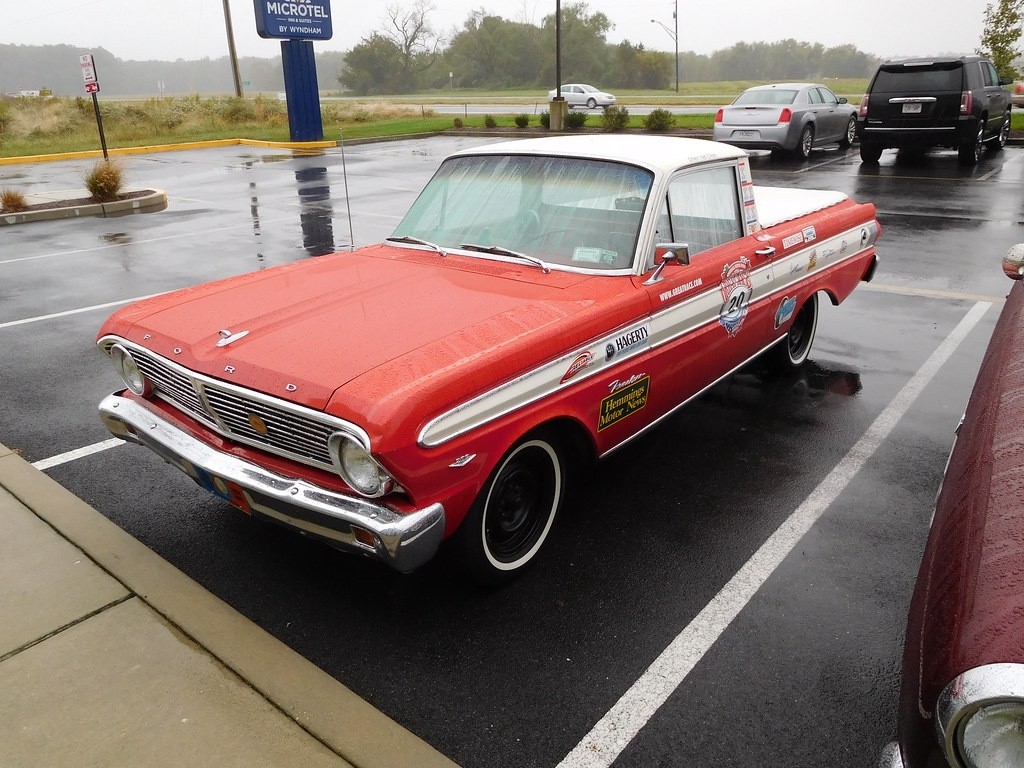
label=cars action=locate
[712,82,859,162]
[94,132,882,587]
[876,239,1023,768]
[548,84,616,110]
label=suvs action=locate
[855,54,1012,171]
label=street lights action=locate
[651,19,680,93]
